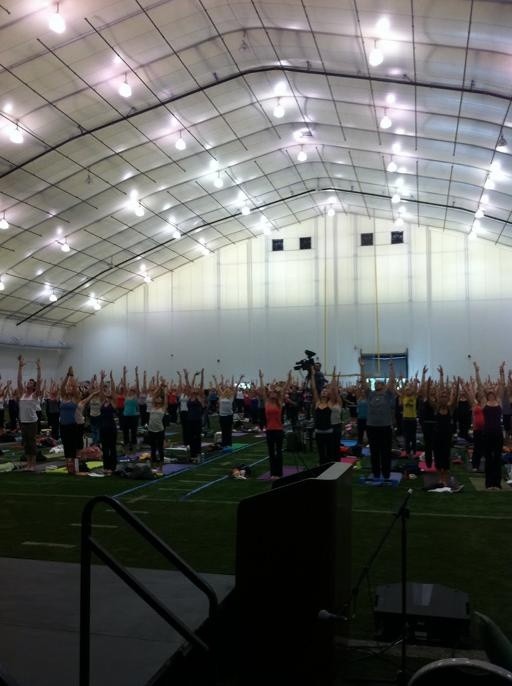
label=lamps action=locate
[213,170,272,237]
[380,108,391,128]
[49,2,67,34]
[173,225,180,239]
[10,122,24,143]
[202,243,210,257]
[85,170,93,185]
[387,141,412,228]
[60,237,71,254]
[239,29,251,54]
[367,37,383,67]
[144,272,152,284]
[297,145,308,163]
[93,299,101,310]
[119,72,132,98]
[1,279,5,291]
[315,179,337,216]
[467,162,500,238]
[106,256,115,269]
[49,289,57,302]
[2,210,9,230]
[135,199,144,217]
[272,98,285,119]
[496,132,508,151]
[175,130,186,150]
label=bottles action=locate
[74,458,80,473]
[439,467,445,481]
[200,452,206,464]
[464,452,467,464]
[262,425,268,441]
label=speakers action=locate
[236,461,353,629]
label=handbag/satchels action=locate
[132,465,153,480]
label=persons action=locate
[0,348,511,493]
